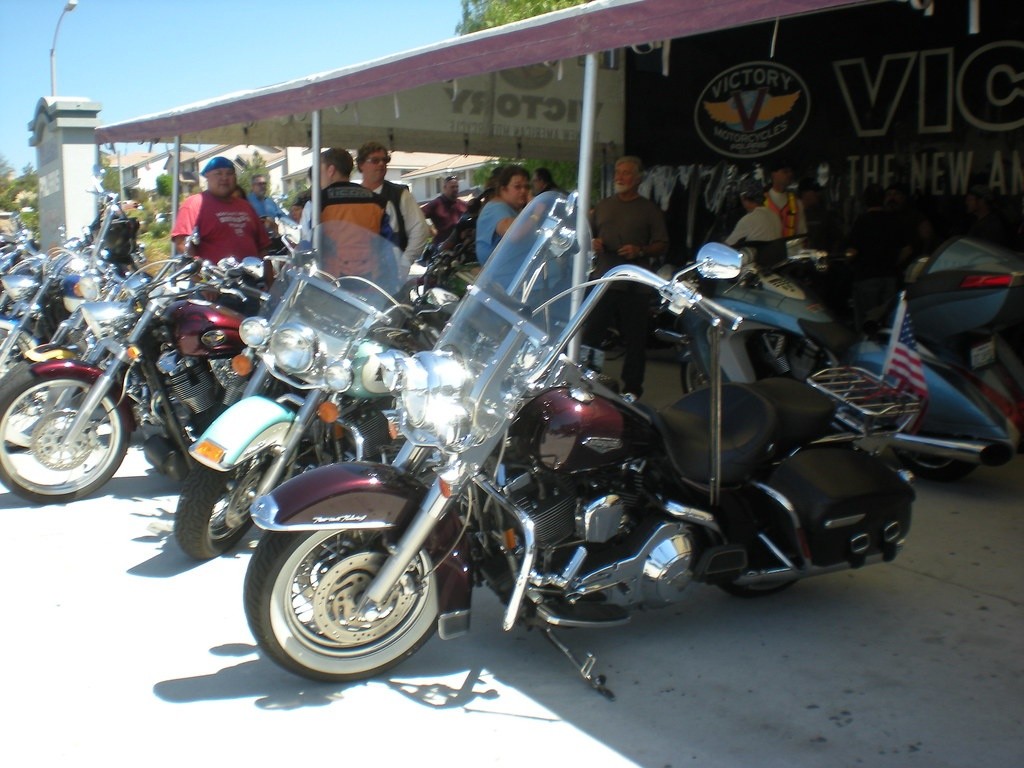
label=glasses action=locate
[255,182,266,185]
[367,156,390,164]
[446,176,457,181]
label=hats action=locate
[200,157,233,176]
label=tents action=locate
[96,1,1024,368]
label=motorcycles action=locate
[0,191,271,504]
[652,236,1024,483]
[173,221,460,562]
[243,189,927,704]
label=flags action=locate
[881,297,931,437]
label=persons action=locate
[356,141,428,310]
[289,166,313,224]
[248,173,285,225]
[290,203,304,223]
[297,146,402,297]
[726,164,950,292]
[420,164,596,283]
[584,153,668,400]
[169,157,266,317]
[231,185,248,200]
[476,164,562,335]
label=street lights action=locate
[50,0,78,97]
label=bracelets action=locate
[591,238,595,242]
[530,215,539,221]
